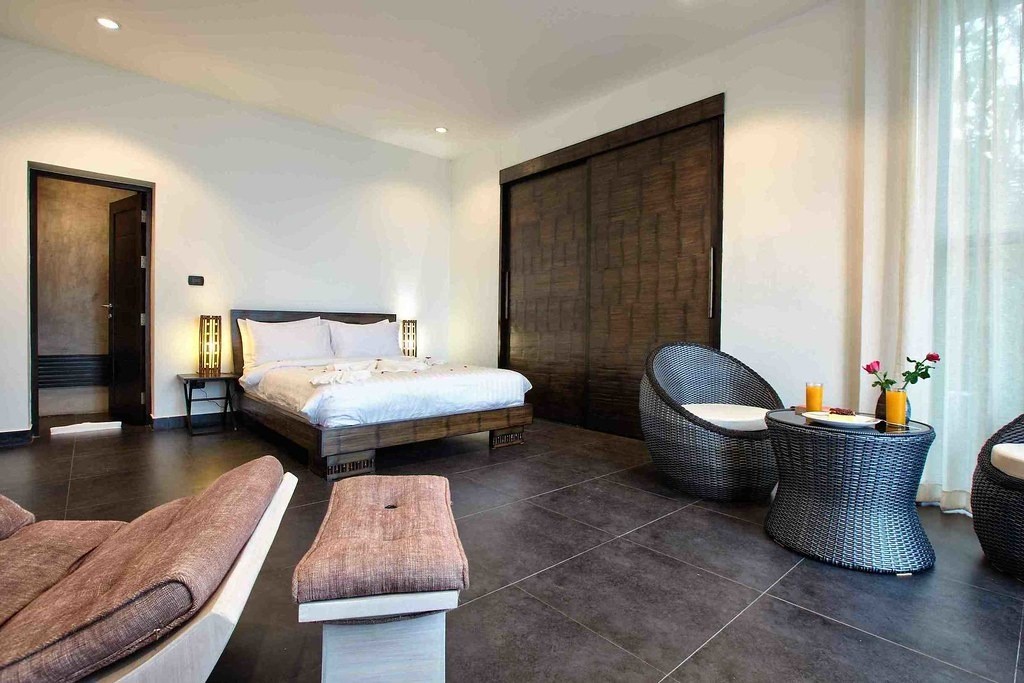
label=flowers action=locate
[861,352,940,390]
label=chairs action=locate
[0,456,297,683]
[970,414,1024,574]
[639,341,784,499]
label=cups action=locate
[886,388,906,427]
[806,382,823,412]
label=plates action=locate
[802,412,880,428]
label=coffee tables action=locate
[764,406,937,576]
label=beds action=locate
[229,309,535,482]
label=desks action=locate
[177,374,241,437]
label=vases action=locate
[875,390,910,431]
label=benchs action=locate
[291,474,469,683]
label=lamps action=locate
[199,314,222,379]
[402,319,417,357]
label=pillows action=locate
[235,316,321,369]
[323,318,390,360]
[329,321,402,360]
[245,316,332,367]
